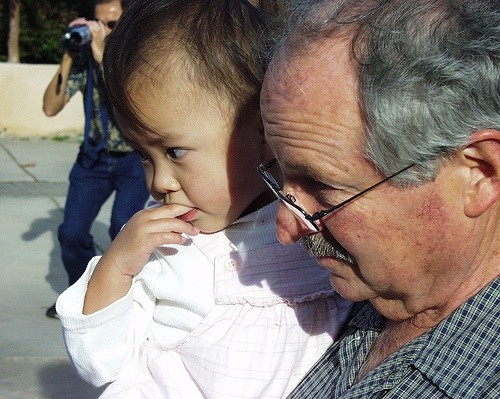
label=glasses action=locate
[255,160,416,233]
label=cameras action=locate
[65,24,92,48]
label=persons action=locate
[56,1,352,399]
[42,1,150,317]
[258,0,499,399]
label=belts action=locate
[101,149,130,157]
